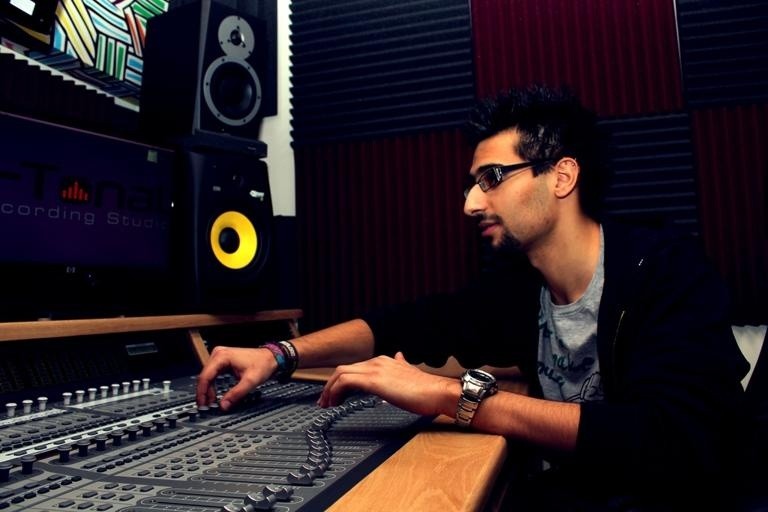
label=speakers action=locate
[139,0,268,159]
[186,151,274,315]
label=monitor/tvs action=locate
[0,108,180,269]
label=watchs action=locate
[453,368,499,429]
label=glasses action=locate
[463,157,557,199]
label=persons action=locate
[195,79,751,512]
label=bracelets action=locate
[257,340,298,385]
